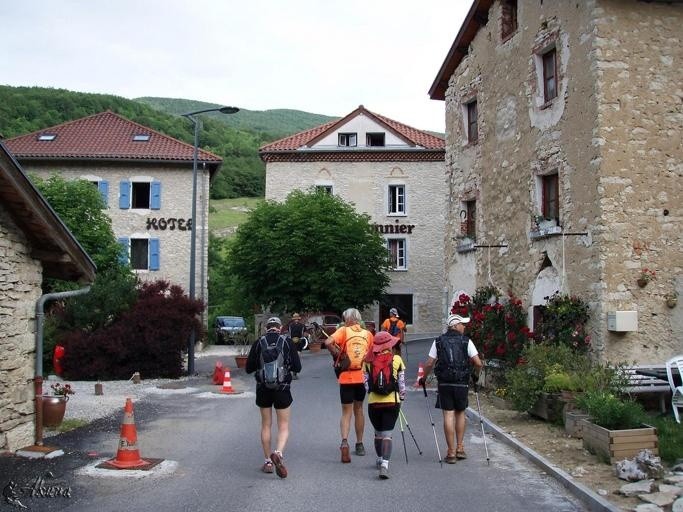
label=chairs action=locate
[665,354,683,426]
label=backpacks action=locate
[258,334,289,392]
[440,331,474,387]
[363,352,398,395]
[339,327,368,372]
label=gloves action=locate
[419,377,425,385]
[471,373,479,383]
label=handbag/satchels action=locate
[333,326,351,378]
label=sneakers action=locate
[264,462,273,473]
[456,448,468,460]
[340,443,352,463]
[269,453,288,478]
[444,455,456,464]
[377,458,382,469]
[292,375,298,380]
[379,466,390,479]
[355,446,366,456]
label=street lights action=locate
[180,105,241,377]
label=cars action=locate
[281,311,376,345]
[212,315,249,344]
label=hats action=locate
[446,315,471,326]
[291,313,302,321]
[372,332,400,353]
[267,317,282,326]
[390,308,401,318]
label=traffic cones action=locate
[100,396,151,470]
[219,367,234,392]
[413,363,428,386]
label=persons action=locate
[381,308,407,358]
[289,313,307,380]
[417,314,482,463]
[243,316,303,477]
[322,308,372,463]
[361,331,407,478]
